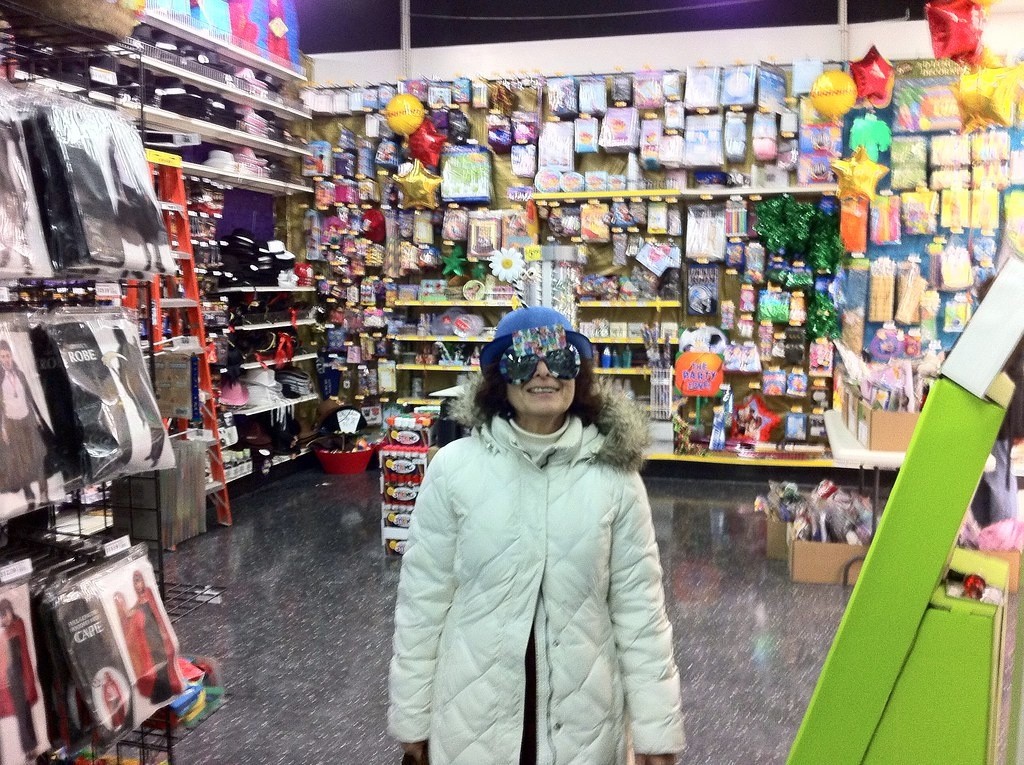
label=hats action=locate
[201,144,273,179]
[257,71,279,92]
[193,49,227,71]
[311,399,341,429]
[240,411,319,455]
[131,24,157,46]
[221,232,297,288]
[154,76,280,141]
[179,44,197,62]
[247,364,311,396]
[234,67,256,84]
[155,33,186,56]
[480,306,594,386]
[218,379,249,406]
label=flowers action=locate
[488,248,525,282]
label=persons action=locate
[388,306,686,765]
[0,340,60,511]
[0,599,38,761]
[114,571,181,705]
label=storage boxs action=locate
[842,391,921,450]
[789,539,871,586]
[767,520,792,560]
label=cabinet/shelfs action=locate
[116,5,322,494]
[394,182,834,467]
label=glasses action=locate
[500,343,582,385]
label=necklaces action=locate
[7,375,18,398]
